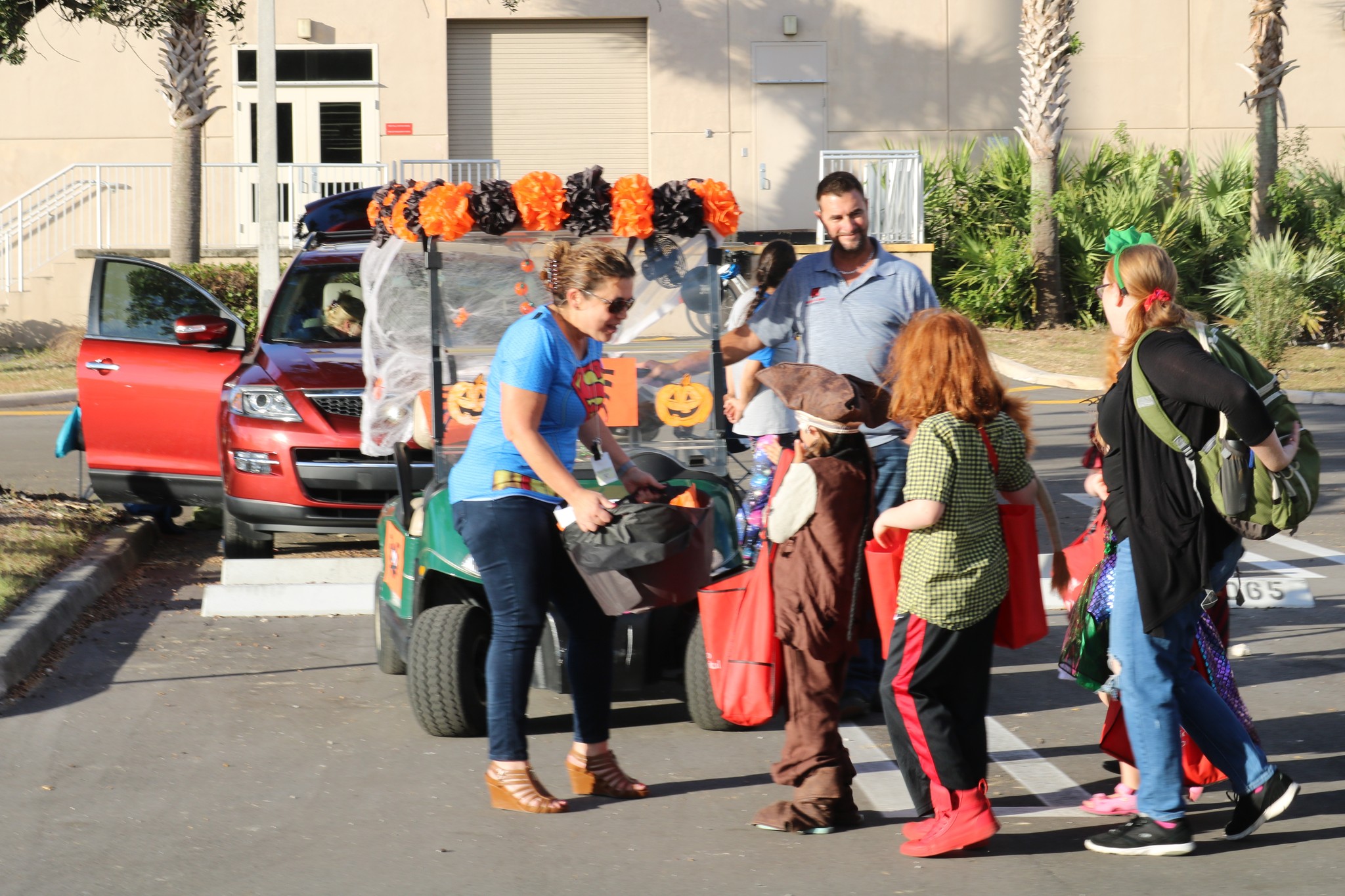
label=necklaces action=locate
[557,307,603,461]
[837,247,876,275]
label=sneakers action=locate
[899,779,999,855]
[1085,815,1195,857]
[900,815,936,841]
[1225,767,1301,840]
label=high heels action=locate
[567,747,648,799]
[485,761,568,813]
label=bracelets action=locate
[615,460,638,480]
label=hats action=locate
[757,363,869,434]
[338,294,365,320]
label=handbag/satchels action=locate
[697,448,794,725]
[863,424,1050,659]
[1053,501,1108,611]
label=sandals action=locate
[1080,784,1141,817]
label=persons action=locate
[448,241,664,814]
[286,293,366,343]
[636,172,940,724]
[1058,226,1300,857]
[871,311,1041,857]
[751,362,892,834]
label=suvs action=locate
[77,227,434,561]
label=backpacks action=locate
[1128,324,1319,540]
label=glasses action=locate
[1096,283,1124,299]
[569,284,636,312]
